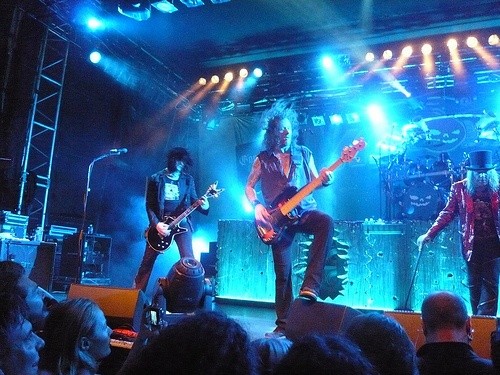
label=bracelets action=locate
[250,199,260,209]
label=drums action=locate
[429,159,452,186]
[401,182,448,223]
[403,161,429,186]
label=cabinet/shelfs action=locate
[59,234,113,279]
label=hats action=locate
[464,151,497,170]
[169,147,192,164]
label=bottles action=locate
[88,224,94,235]
[34,227,42,242]
[363,217,385,225]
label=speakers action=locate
[287,299,364,343]
[67,283,149,332]
[0,238,57,293]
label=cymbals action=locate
[377,153,403,164]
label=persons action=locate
[244,104,336,338]
[415,150,500,320]
[0,260,497,375]
[131,147,210,294]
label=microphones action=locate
[109,148,128,154]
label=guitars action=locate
[145,177,226,254]
[254,138,366,244]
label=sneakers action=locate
[265,326,286,339]
[299,286,318,301]
